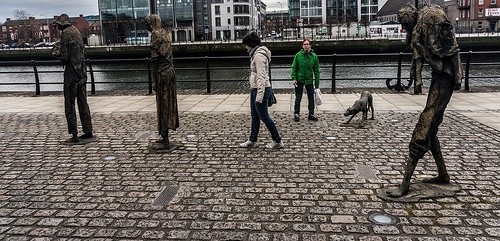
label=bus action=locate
[271,31,275,35]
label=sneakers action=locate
[240,140,258,147]
[265,140,284,149]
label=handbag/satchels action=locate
[314,89,322,104]
[268,92,276,107]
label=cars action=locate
[266,33,271,37]
[0,42,56,48]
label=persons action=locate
[240,34,284,148]
[386,4,455,197]
[53,14,93,142]
[144,14,179,149]
[291,39,320,121]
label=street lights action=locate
[40,14,50,42]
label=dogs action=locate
[343,92,375,126]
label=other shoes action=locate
[308,114,318,121]
[294,114,300,120]
